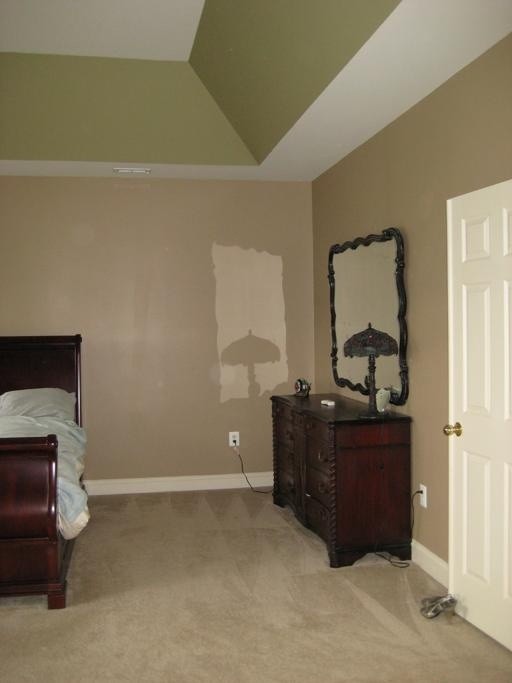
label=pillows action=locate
[0,388,77,419]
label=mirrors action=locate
[328,227,408,406]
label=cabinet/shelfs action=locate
[269,393,414,568]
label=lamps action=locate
[344,322,399,420]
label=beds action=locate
[0,335,82,609]
